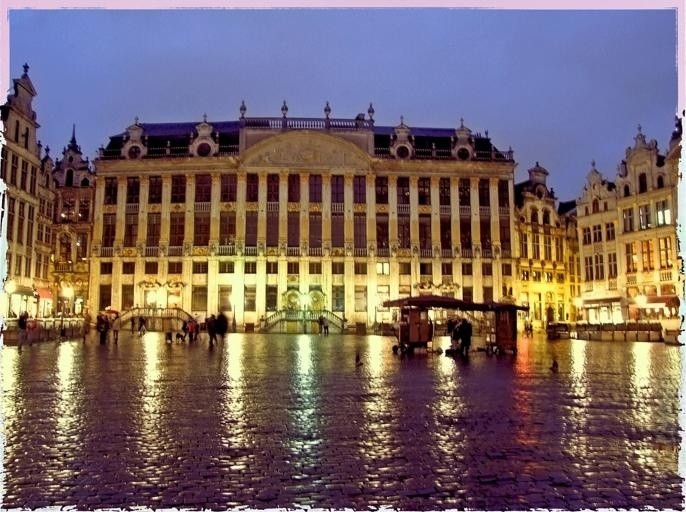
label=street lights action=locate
[298,293,309,334]
[371,292,381,322]
[227,292,243,333]
[59,284,74,343]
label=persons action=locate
[97,312,227,344]
[319,314,323,333]
[323,317,329,334]
[18,312,28,350]
[458,318,473,364]
